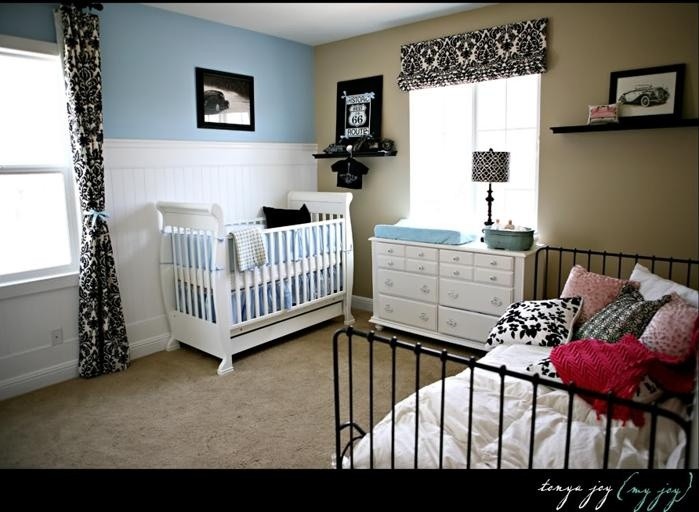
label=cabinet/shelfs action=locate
[367,235,548,352]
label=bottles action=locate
[504,220,516,230]
[491,218,503,229]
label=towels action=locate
[230,227,272,273]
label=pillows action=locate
[559,264,640,320]
[261,204,312,229]
[638,290,699,365]
[572,282,674,345]
[484,296,584,349]
[625,262,699,308]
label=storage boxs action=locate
[480,222,536,253]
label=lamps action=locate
[472,147,511,245]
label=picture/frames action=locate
[194,66,256,132]
[609,62,687,118]
[337,75,383,145]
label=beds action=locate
[152,190,355,375]
[331,245,698,470]
